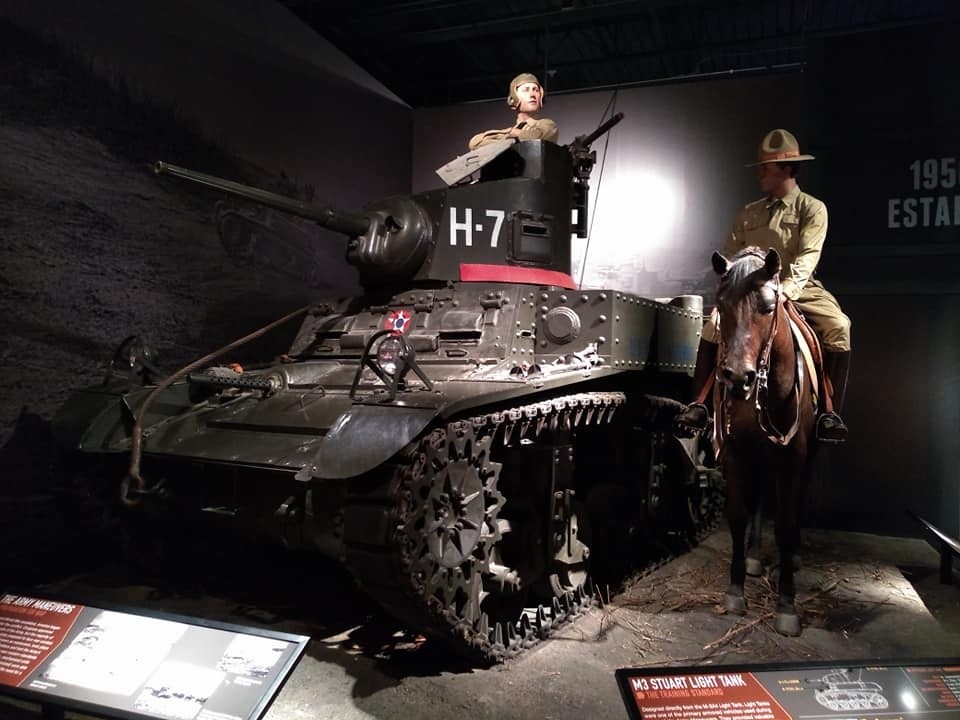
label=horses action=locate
[710,244,820,636]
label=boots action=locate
[678,337,719,427]
[820,351,849,437]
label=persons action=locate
[675,129,851,439]
[468,73,559,151]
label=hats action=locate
[744,129,815,167]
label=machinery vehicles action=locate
[72,106,726,667]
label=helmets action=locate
[507,73,545,111]
